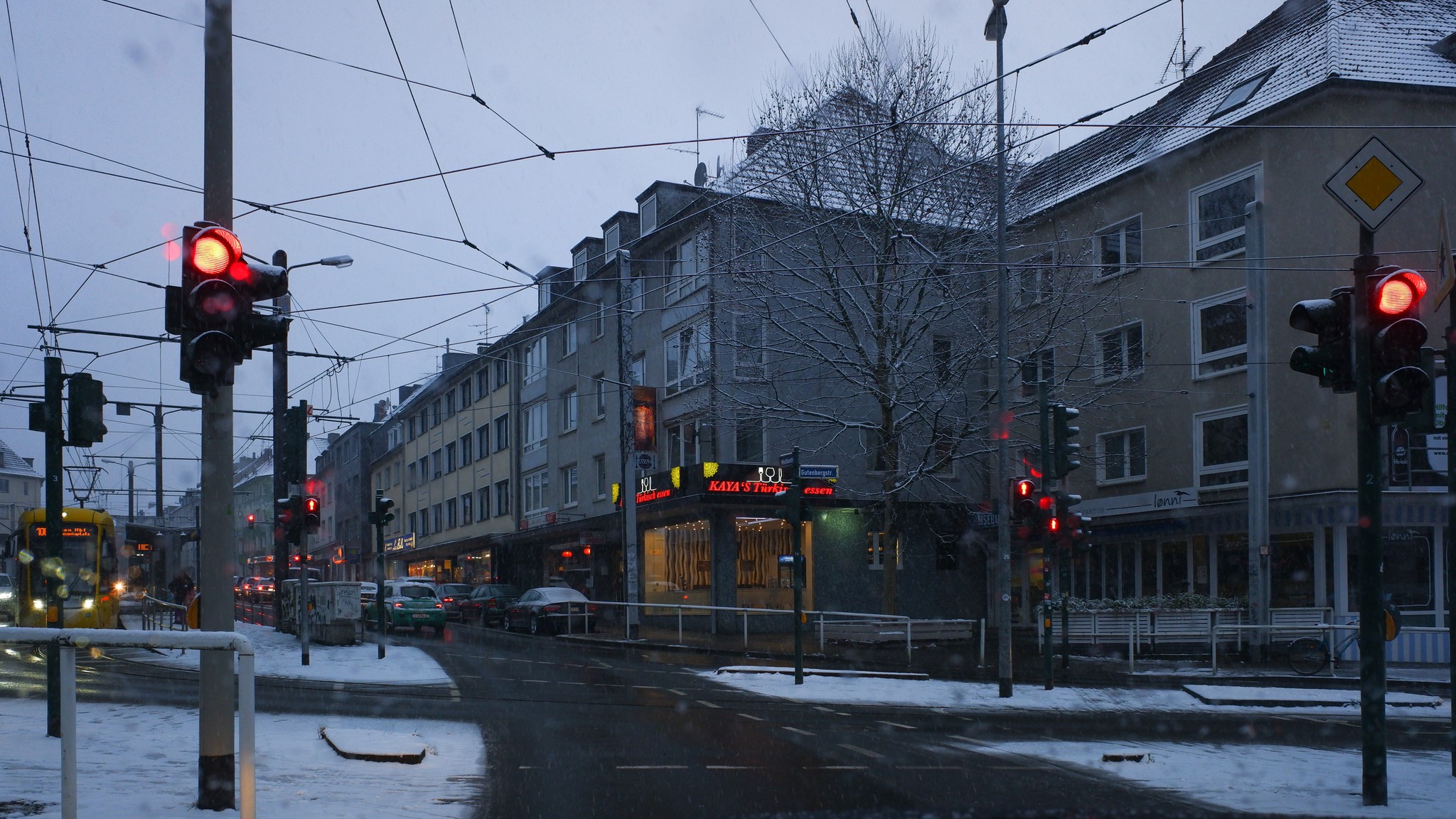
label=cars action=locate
[234,576,276,603]
[0,573,18,621]
[365,581,446,635]
[359,575,473,622]
[502,587,598,637]
[458,583,524,628]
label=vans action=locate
[290,568,322,582]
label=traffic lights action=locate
[1288,291,1357,395]
[248,515,254,530]
[1013,476,1036,519]
[243,260,294,363]
[177,225,242,393]
[1364,266,1438,427]
[1053,399,1081,477]
[277,493,321,546]
[774,487,793,523]
[1072,515,1093,554]
[1055,490,1083,540]
[376,495,395,527]
[281,400,306,484]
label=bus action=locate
[3,508,120,629]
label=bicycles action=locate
[1287,620,1360,675]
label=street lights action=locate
[100,460,158,523]
[270,249,354,632]
[131,401,202,603]
